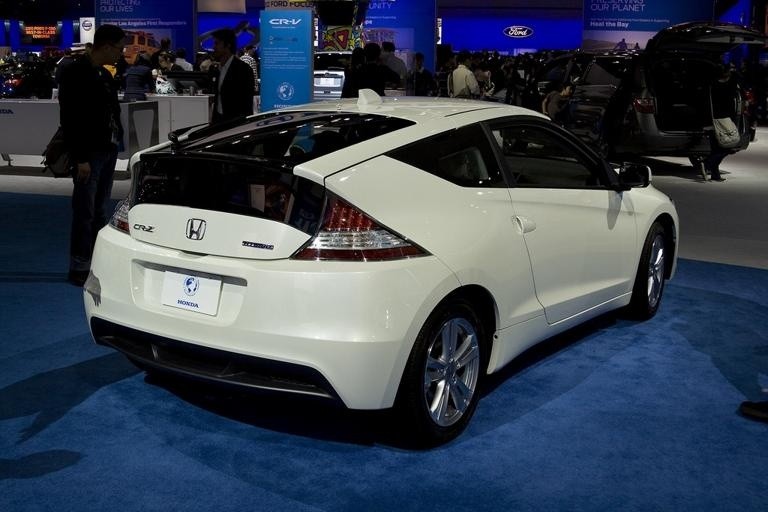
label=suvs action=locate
[540,18,768,156]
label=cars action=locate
[79,92,681,453]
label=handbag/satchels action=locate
[712,116,740,148]
[44,127,74,179]
[155,77,176,95]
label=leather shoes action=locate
[739,400,768,424]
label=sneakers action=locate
[700,159,726,182]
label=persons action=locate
[697,62,738,182]
[0,24,259,123]
[340,42,585,145]
[614,38,627,49]
[635,42,640,50]
[56,25,125,288]
